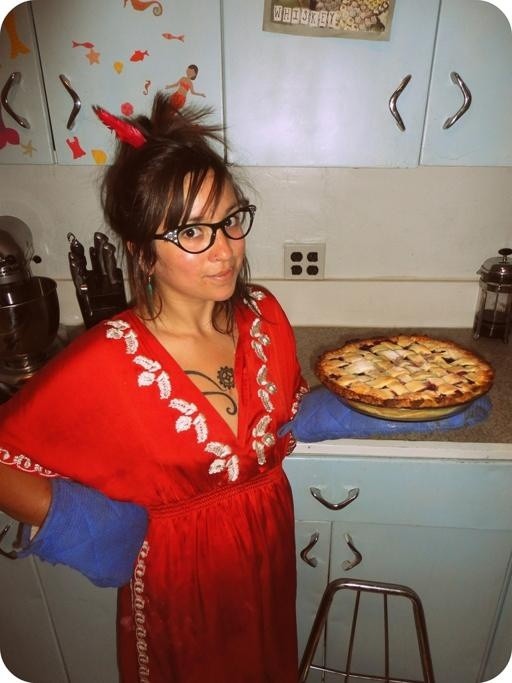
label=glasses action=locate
[151,205,256,254]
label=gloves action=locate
[0,480,148,587]
[277,385,492,443]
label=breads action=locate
[315,334,496,420]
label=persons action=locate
[1,101,306,678]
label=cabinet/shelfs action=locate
[282,459,511,682]
[0,1,225,165]
[1,509,119,683]
[220,0,511,168]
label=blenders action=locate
[0,217,69,386]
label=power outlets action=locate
[283,241,325,281]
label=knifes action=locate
[68,231,118,293]
